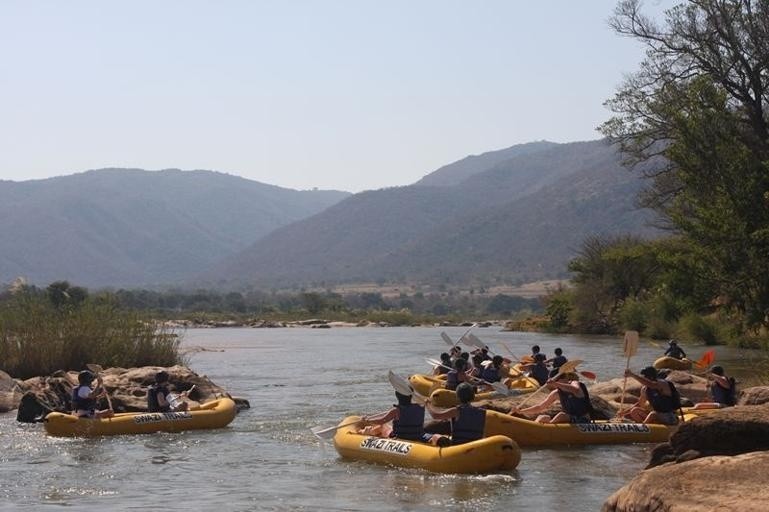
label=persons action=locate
[511,367,594,424]
[358,385,424,442]
[623,365,681,425]
[424,382,486,448]
[72,370,114,419]
[663,339,686,360]
[432,343,567,393]
[147,370,188,413]
[694,365,736,409]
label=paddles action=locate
[620,331,639,404]
[509,358,584,416]
[440,332,461,354]
[87,364,112,409]
[684,350,715,370]
[576,371,597,380]
[462,331,540,388]
[316,411,387,439]
[426,358,509,397]
[388,369,425,402]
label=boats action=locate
[42,396,239,436]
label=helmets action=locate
[156,371,168,383]
[641,366,657,377]
[669,340,677,344]
[78,370,95,384]
[396,387,414,403]
[457,383,475,400]
